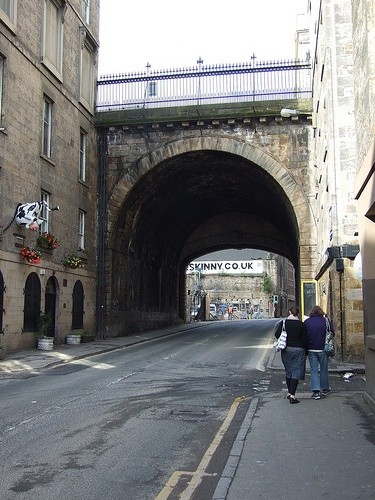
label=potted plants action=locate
[66,329,83,345]
[35,314,54,351]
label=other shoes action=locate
[323,390,328,395]
[287,394,300,404]
[311,393,320,399]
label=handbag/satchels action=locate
[276,320,287,352]
[324,317,335,357]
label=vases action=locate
[68,265,79,269]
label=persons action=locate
[274,306,308,404]
[303,306,335,400]
[228,305,233,320]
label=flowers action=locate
[37,230,60,249]
[19,248,43,264]
[61,252,84,269]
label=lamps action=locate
[281,108,313,117]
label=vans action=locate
[192,304,217,320]
